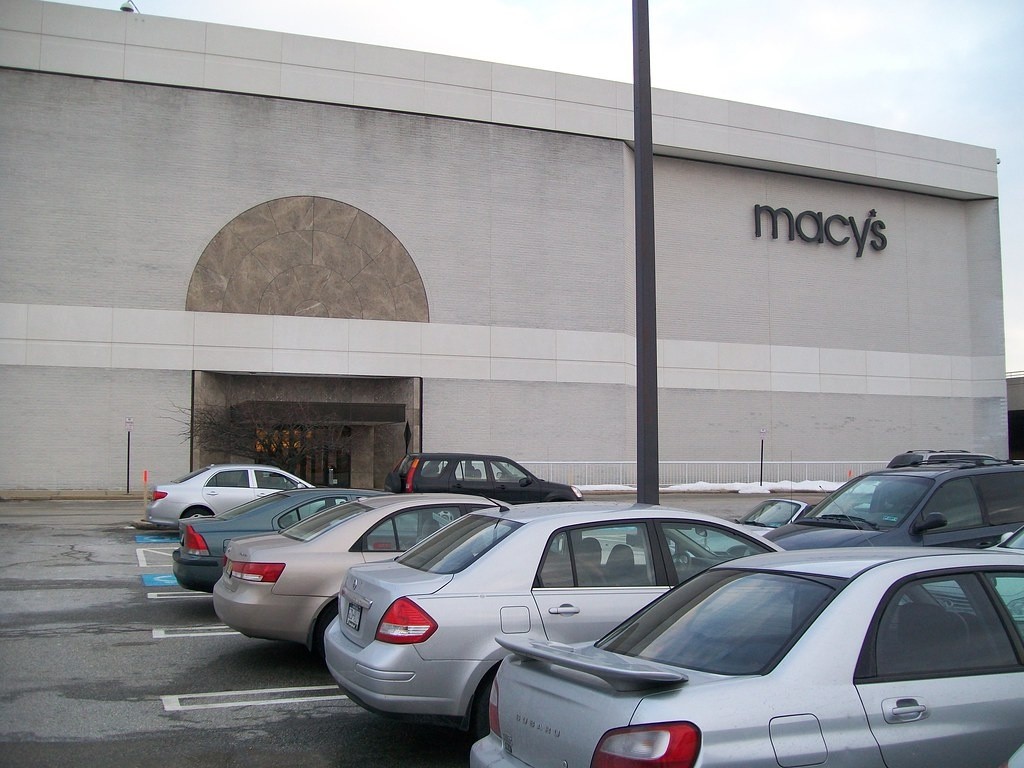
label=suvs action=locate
[383,451,585,507]
[743,447,1024,557]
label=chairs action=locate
[606,545,635,579]
[577,537,602,567]
[471,466,482,479]
[898,602,999,666]
[415,519,440,543]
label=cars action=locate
[174,487,455,595]
[698,498,820,551]
[212,491,511,658]
[920,525,1024,628]
[322,500,791,742]
[468,544,1024,768]
[140,462,353,533]
